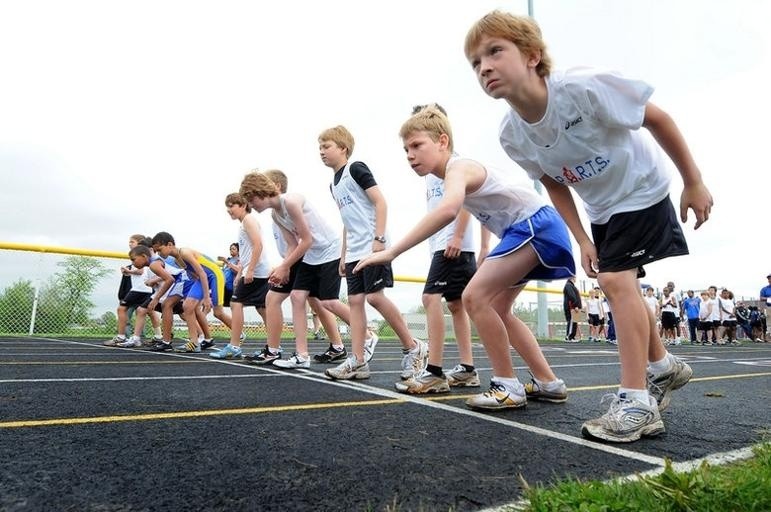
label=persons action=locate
[353,108,576,411]
[465,16,712,442]
[564,270,771,355]
[395,102,480,395]
[317,125,430,381]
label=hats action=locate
[766,273,771,278]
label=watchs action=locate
[375,235,387,243]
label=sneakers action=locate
[253,344,285,356]
[582,397,668,445]
[401,338,430,380]
[230,330,246,347]
[444,363,480,388]
[267,353,313,374]
[357,328,376,363]
[465,379,529,411]
[663,335,771,350]
[101,331,214,358]
[560,333,619,346]
[207,344,243,361]
[313,333,325,341]
[313,343,348,364]
[393,372,453,397]
[242,345,283,364]
[641,358,695,410]
[519,376,568,403]
[325,359,371,382]
